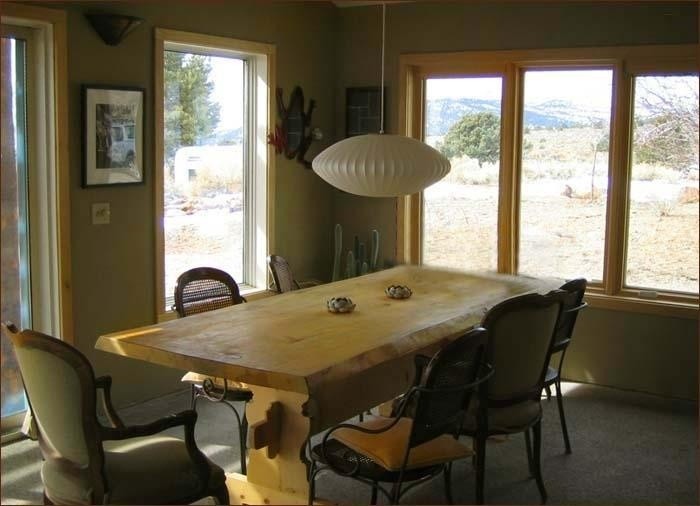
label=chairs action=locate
[385,287,571,504]
[0,317,234,504]
[297,324,492,505]
[484,277,589,469]
[170,265,257,478]
[266,255,315,294]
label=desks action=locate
[88,262,565,506]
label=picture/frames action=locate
[80,84,146,187]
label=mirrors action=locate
[267,86,316,170]
[346,85,385,145]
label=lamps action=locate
[311,0,451,199]
[85,13,147,45]
